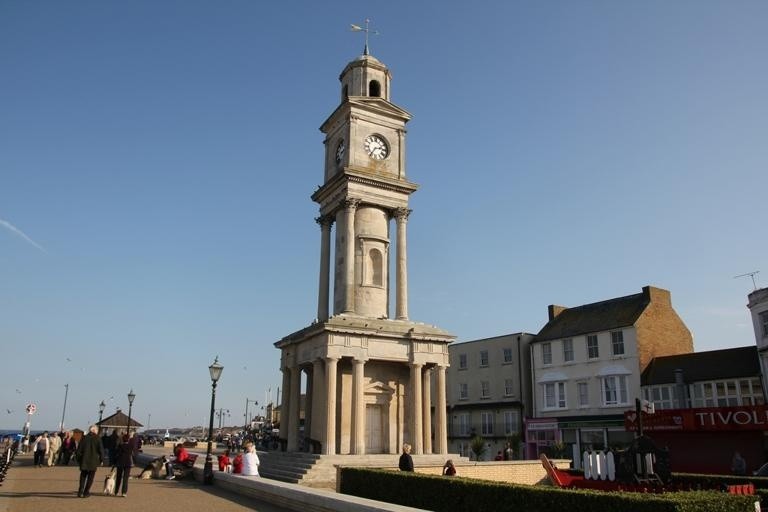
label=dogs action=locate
[103,475,115,496]
[138,455,167,480]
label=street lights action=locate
[127,389,136,434]
[214,407,230,428]
[204,355,224,486]
[246,398,259,423]
[99,400,106,422]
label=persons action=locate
[504,442,514,460]
[21,424,144,497]
[216,430,268,476]
[443,459,456,476]
[399,443,415,472]
[166,443,190,479]
[164,446,179,475]
[495,451,502,460]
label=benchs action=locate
[173,452,198,479]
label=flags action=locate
[641,400,655,414]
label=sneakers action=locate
[33,463,55,469]
[77,491,128,499]
[166,475,176,480]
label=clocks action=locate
[336,132,392,165]
